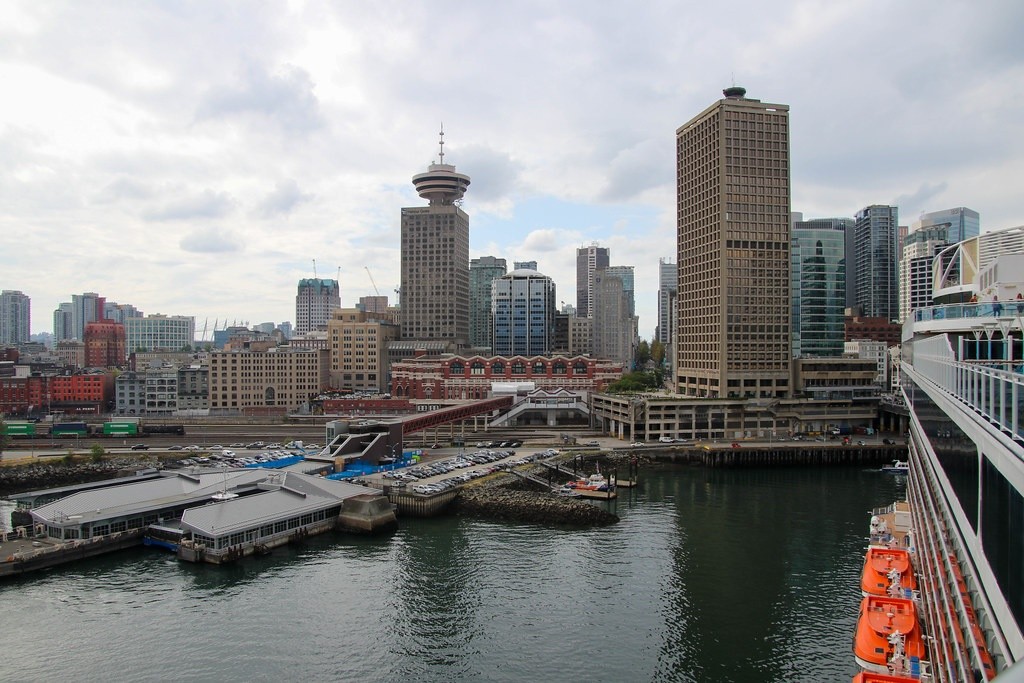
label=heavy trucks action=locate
[856,426,874,436]
[831,428,852,435]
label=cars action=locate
[882,438,896,445]
[167,438,322,469]
[341,437,560,495]
[777,437,785,441]
[790,436,800,441]
[858,440,866,446]
[586,440,600,446]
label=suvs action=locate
[630,441,644,448]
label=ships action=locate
[850,227,1024,683]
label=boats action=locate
[859,544,918,601]
[881,459,909,475]
[851,670,924,683]
[852,595,929,676]
[565,460,614,492]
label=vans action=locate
[658,436,674,443]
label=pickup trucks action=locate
[131,443,150,450]
